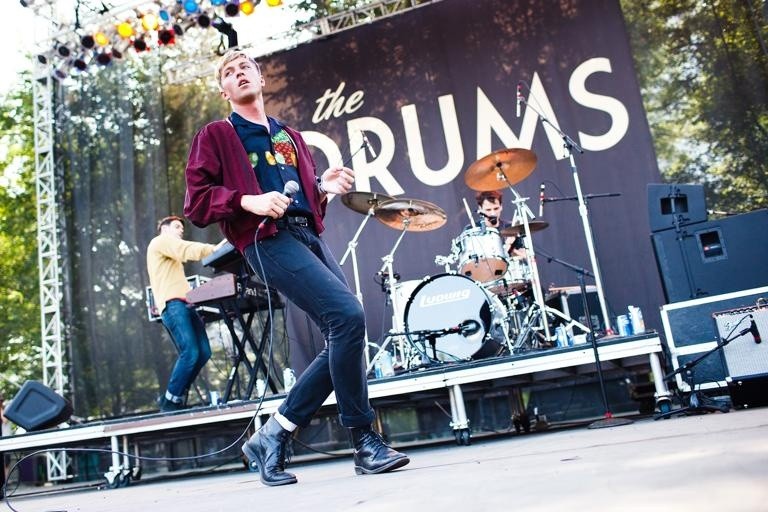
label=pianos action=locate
[185,241,281,313]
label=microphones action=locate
[539,183,546,217]
[257,179,300,232]
[516,83,522,117]
[360,129,377,159]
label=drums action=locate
[455,226,509,282]
[403,273,510,364]
[486,256,529,295]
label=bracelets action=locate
[318,186,327,195]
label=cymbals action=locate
[465,149,537,192]
[500,221,549,236]
[375,198,448,232]
[341,191,396,217]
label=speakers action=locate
[4,380,73,432]
[646,182,708,233]
[650,208,768,302]
[751,315,761,344]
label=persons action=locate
[145,215,220,412]
[465,189,546,356]
[0,404,12,484]
[183,49,412,485]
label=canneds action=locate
[616,315,632,336]
[211,391,220,406]
[375,360,383,378]
[554,328,565,348]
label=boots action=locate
[241,413,297,486]
[345,423,409,476]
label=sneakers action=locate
[158,394,188,412]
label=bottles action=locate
[380,351,394,377]
[284,368,294,394]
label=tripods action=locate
[486,172,600,355]
[651,328,754,420]
[364,223,428,378]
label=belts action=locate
[276,212,316,228]
